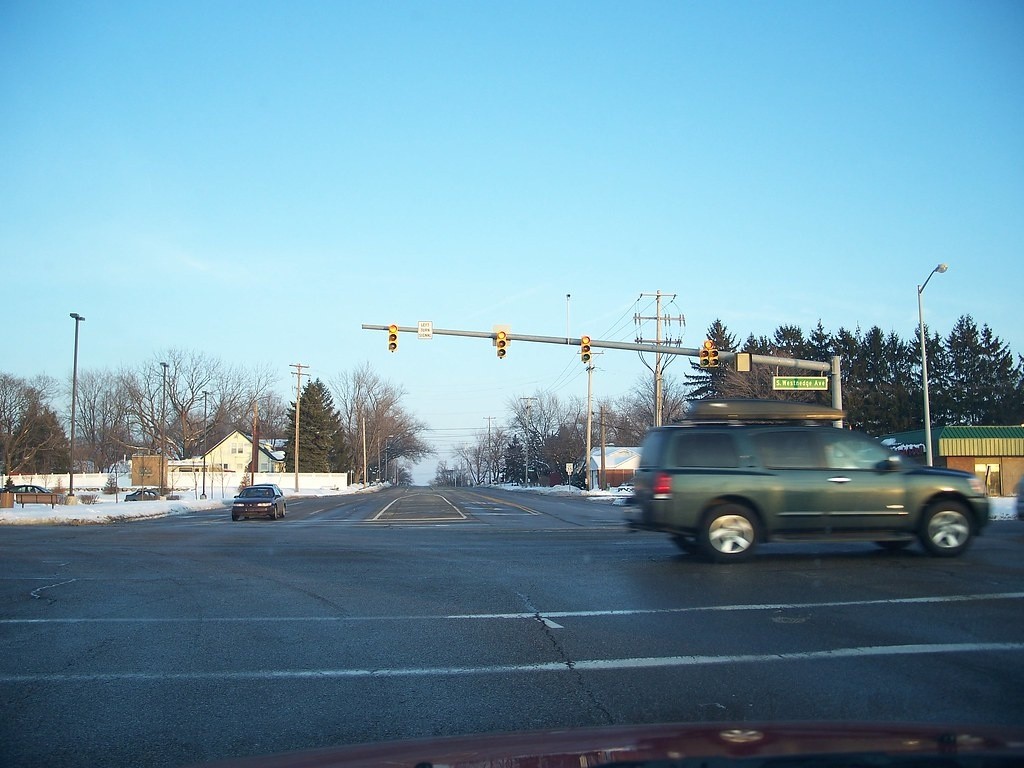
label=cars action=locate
[124,489,160,502]
[231,484,287,520]
[0,484,66,502]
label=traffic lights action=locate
[580,336,592,362]
[388,324,399,353]
[709,349,720,368]
[699,349,710,368]
[496,330,507,358]
[704,339,714,349]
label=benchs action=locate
[16,494,59,509]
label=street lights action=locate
[917,263,949,468]
[202,390,210,496]
[68,311,85,496]
[160,361,170,497]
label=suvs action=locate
[625,396,991,562]
